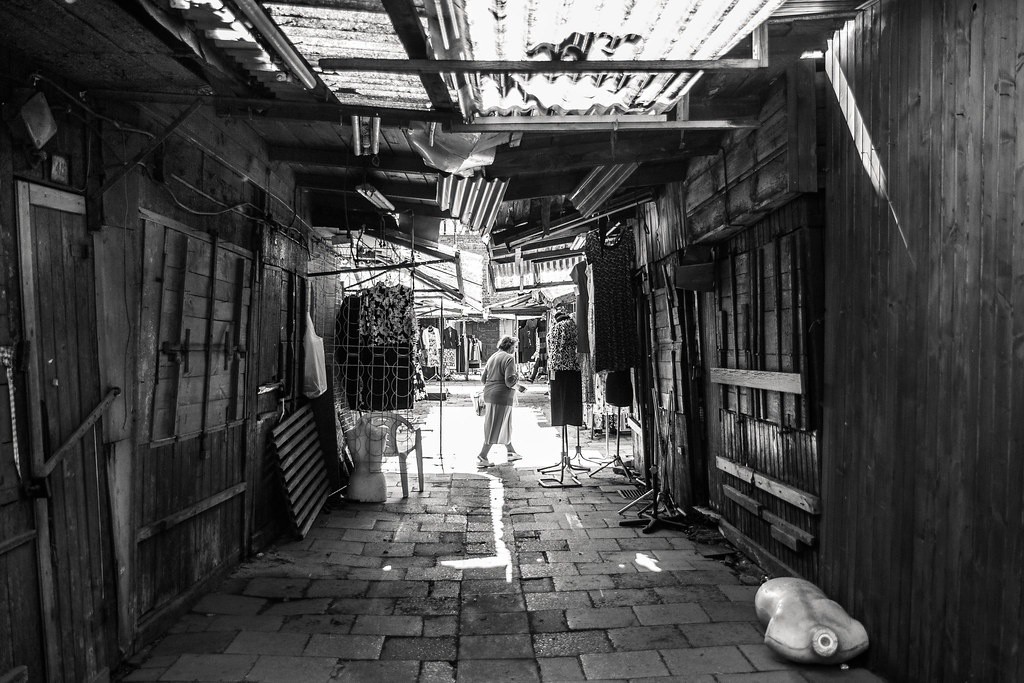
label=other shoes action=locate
[506,451,523,463]
[476,454,495,470]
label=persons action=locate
[476,337,527,467]
[545,313,583,427]
[755,576,869,664]
[344,418,389,502]
[525,350,541,383]
[424,325,459,369]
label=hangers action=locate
[586,214,631,238]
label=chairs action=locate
[353,412,424,499]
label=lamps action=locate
[355,182,396,212]
[351,113,381,156]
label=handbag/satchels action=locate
[473,391,486,418]
